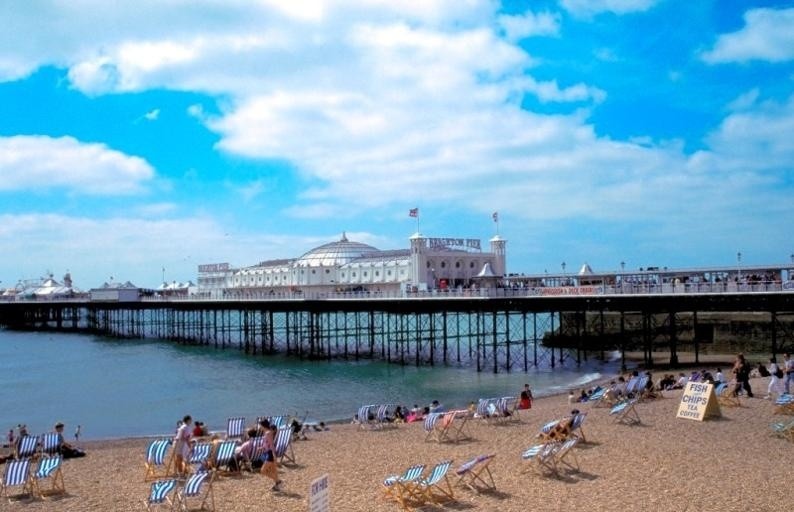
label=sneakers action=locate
[268,481,283,492]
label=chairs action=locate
[239,436,266,472]
[415,460,456,508]
[177,471,210,510]
[453,451,496,495]
[203,439,238,477]
[354,400,404,429]
[184,443,211,475]
[383,464,427,510]
[23,455,66,501]
[145,439,172,478]
[223,409,319,439]
[471,395,520,427]
[41,432,59,455]
[9,435,37,462]
[539,374,794,443]
[143,479,175,512]
[3,457,32,507]
[423,412,451,446]
[440,409,471,444]
[521,440,559,476]
[269,432,296,468]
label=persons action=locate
[53,421,87,458]
[427,279,546,294]
[7,424,37,459]
[566,351,794,403]
[467,401,477,414]
[74,425,81,443]
[520,383,533,409]
[226,426,257,475]
[192,421,210,438]
[562,272,793,290]
[290,418,328,440]
[172,416,194,480]
[272,424,278,439]
[540,407,580,440]
[255,418,284,493]
[335,286,383,297]
[351,400,445,425]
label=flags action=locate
[409,208,417,217]
[492,212,497,222]
